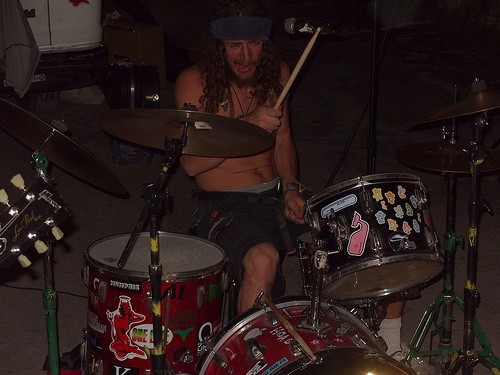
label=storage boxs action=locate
[101,21,168,91]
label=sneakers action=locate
[389,342,434,375]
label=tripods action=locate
[401,116,500,375]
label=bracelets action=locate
[280,183,299,192]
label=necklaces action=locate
[229,82,256,116]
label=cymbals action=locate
[0,97,127,196]
[96,108,275,159]
[395,141,500,174]
[416,90,500,125]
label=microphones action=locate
[283,17,328,35]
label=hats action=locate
[210,16,272,40]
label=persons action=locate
[176,0,307,315]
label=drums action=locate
[194,295,417,375]
[303,173,445,301]
[81,230,231,375]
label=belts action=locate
[193,179,281,206]
